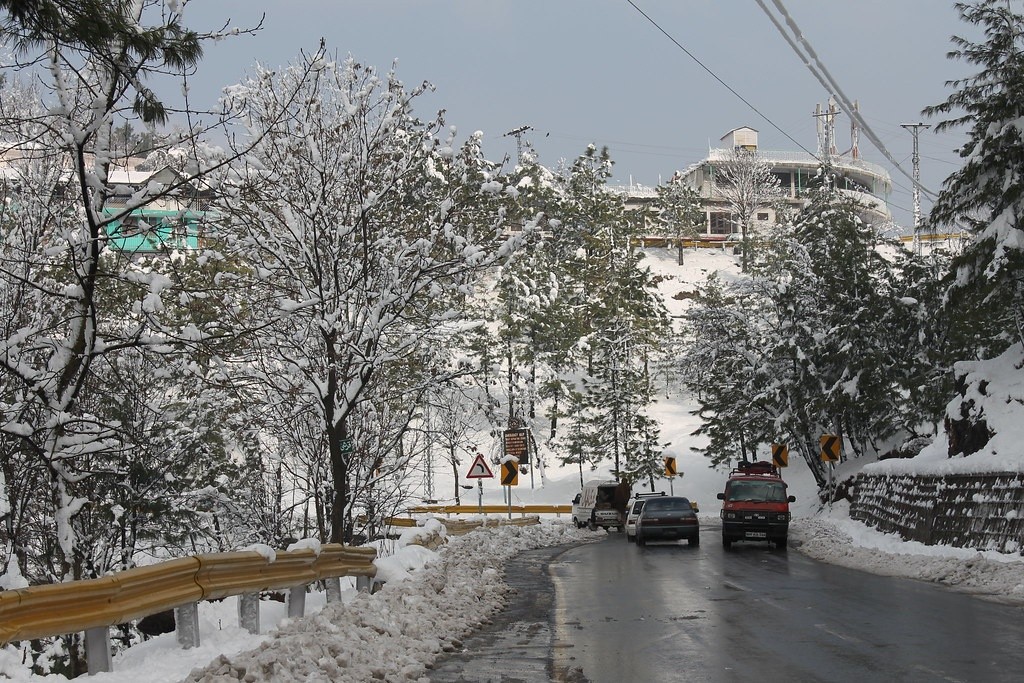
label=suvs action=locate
[625,490,673,543]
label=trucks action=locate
[571,480,629,531]
[716,461,796,551]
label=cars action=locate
[635,497,701,547]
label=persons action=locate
[616,478,631,519]
